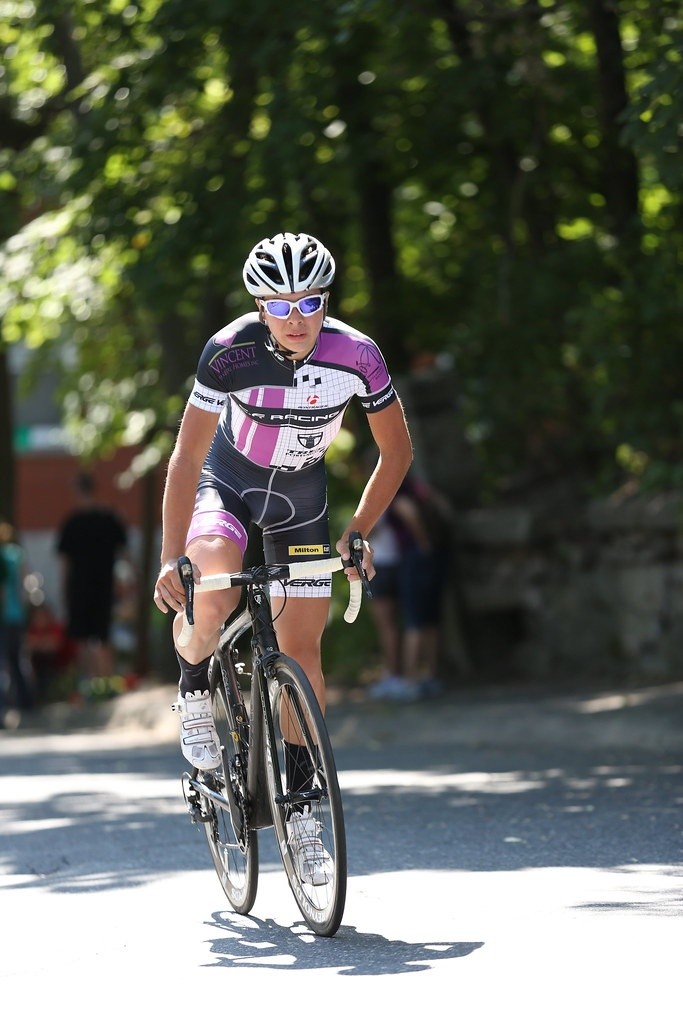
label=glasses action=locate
[256,294,327,320]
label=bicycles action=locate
[176,529,373,937]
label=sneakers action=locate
[172,678,222,771]
[285,805,334,885]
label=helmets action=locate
[242,232,337,297]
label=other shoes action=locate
[370,675,418,702]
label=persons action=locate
[0,520,28,727]
[354,442,448,703]
[154,234,415,882]
[54,473,127,680]
